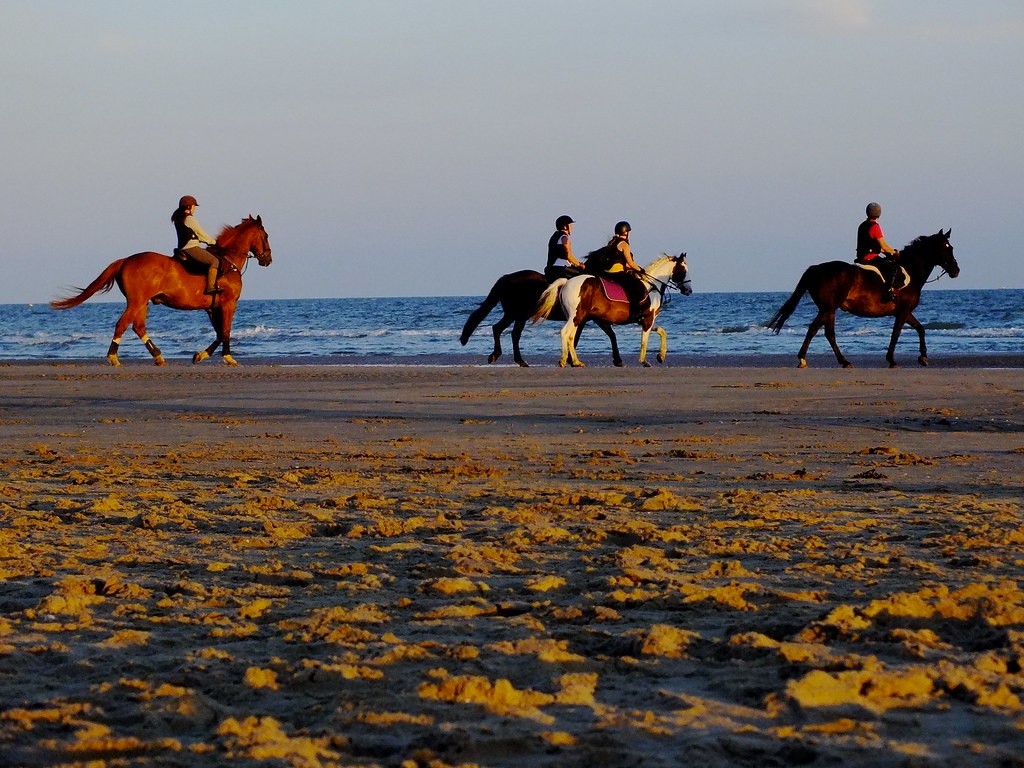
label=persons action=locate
[856,202,900,303]
[543,216,586,284]
[171,195,224,293]
[600,221,651,323]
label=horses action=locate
[43,211,273,372]
[765,225,960,372]
[459,264,627,369]
[530,252,698,369]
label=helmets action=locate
[614,221,632,235]
[179,195,199,210]
[556,215,575,231]
[866,202,882,218]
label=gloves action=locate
[637,265,645,274]
[893,249,899,258]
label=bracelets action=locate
[578,262,584,268]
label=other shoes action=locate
[204,286,224,294]
[880,286,898,304]
